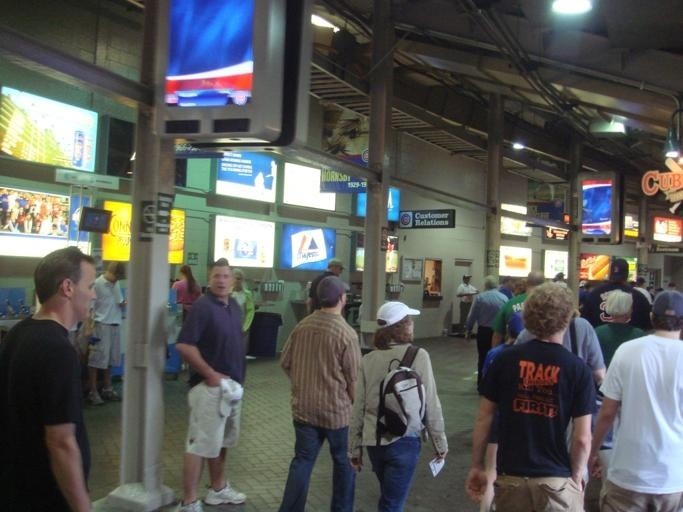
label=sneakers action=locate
[86,385,122,406]
[203,480,247,506]
[176,498,203,512]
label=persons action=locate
[514,309,606,487]
[464,281,597,512]
[279,277,362,512]
[587,289,683,512]
[173,258,247,512]
[347,299,449,511]
[465,270,545,388]
[581,258,677,374]
[458,274,477,336]
[87,262,127,405]
[1,247,103,511]
[305,259,347,315]
[170,265,202,370]
[230,268,256,333]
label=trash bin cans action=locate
[247,312,283,357]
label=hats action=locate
[609,258,630,282]
[375,299,422,330]
[651,289,683,319]
[219,379,244,419]
[316,274,352,305]
[328,258,346,271]
[636,277,646,288]
[463,274,472,280]
[107,261,128,281]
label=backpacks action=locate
[379,359,428,440]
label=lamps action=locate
[663,107,682,160]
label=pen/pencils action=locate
[354,457,361,473]
